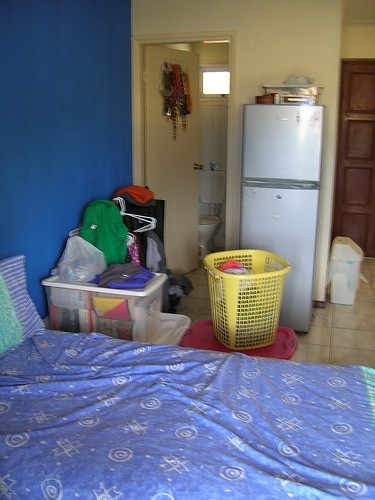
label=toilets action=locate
[198,169,226,261]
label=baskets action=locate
[203,249,290,350]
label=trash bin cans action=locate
[331,235,365,305]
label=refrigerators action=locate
[240,104,323,334]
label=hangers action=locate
[68,197,156,247]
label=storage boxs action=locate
[263,84,324,105]
[43,311,191,345]
[42,272,168,344]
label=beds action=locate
[0,329,375,500]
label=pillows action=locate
[0,255,46,358]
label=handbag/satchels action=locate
[52,235,106,283]
